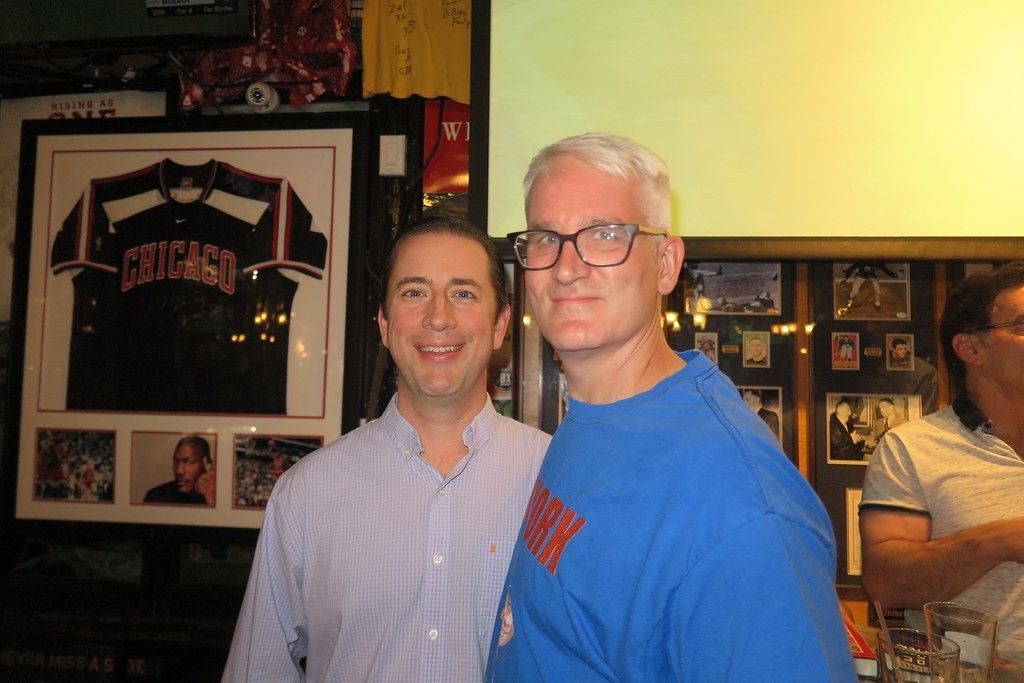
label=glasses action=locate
[507,222,666,270]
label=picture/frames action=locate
[1,110,383,546]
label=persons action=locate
[864,398,908,462]
[838,335,856,361]
[830,399,867,460]
[38,431,113,503]
[145,435,217,506]
[741,389,779,441]
[234,434,292,511]
[482,131,858,683]
[221,215,556,683]
[890,335,910,366]
[688,265,775,312]
[835,259,904,315]
[858,261,1024,683]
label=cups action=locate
[876,628,961,683]
[923,601,999,683]
